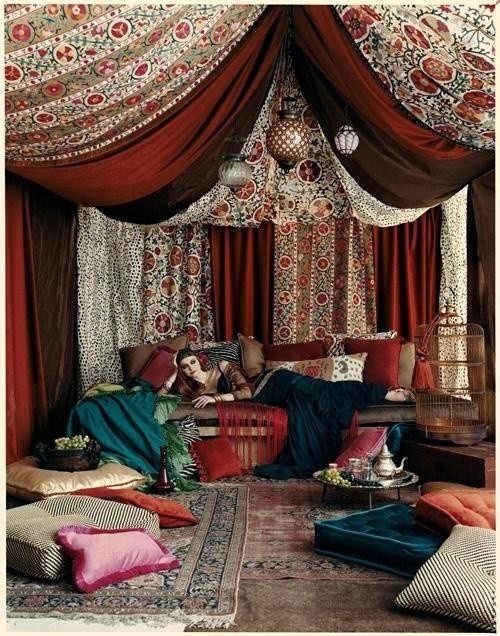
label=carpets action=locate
[239,480,424,584]
[6,482,252,627]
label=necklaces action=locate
[202,372,208,388]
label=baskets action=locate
[34,442,101,470]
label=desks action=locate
[311,462,422,512]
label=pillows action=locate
[417,479,478,497]
[189,435,242,482]
[376,420,454,469]
[162,412,207,481]
[7,448,150,503]
[119,331,418,400]
[391,519,496,632]
[67,486,201,529]
[53,524,183,592]
[333,423,391,469]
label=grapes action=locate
[54,434,97,450]
[319,470,377,487]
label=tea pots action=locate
[365,444,407,480]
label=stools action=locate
[311,501,455,578]
[6,493,161,583]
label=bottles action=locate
[329,456,372,482]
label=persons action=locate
[156,347,417,480]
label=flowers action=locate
[54,432,91,452]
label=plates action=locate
[312,467,420,489]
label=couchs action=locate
[84,385,479,474]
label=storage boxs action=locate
[400,436,495,491]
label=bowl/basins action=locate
[33,437,101,471]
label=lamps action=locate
[262,5,312,176]
[216,118,253,197]
[331,99,362,159]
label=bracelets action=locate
[167,378,175,385]
[213,393,225,401]
